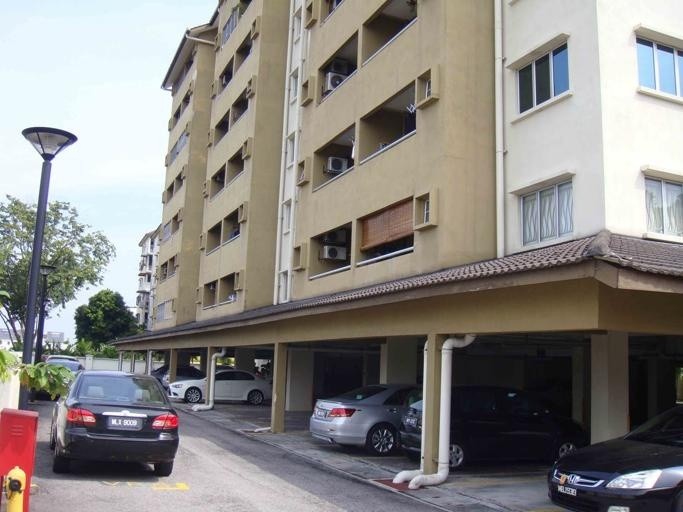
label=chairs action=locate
[86,386,104,399]
[133,389,150,402]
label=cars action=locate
[396,378,587,472]
[304,377,422,458]
[538,400,682,512]
[45,360,272,482]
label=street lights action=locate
[16,121,81,411]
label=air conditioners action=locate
[321,229,347,260]
[326,157,348,174]
[323,58,348,96]
